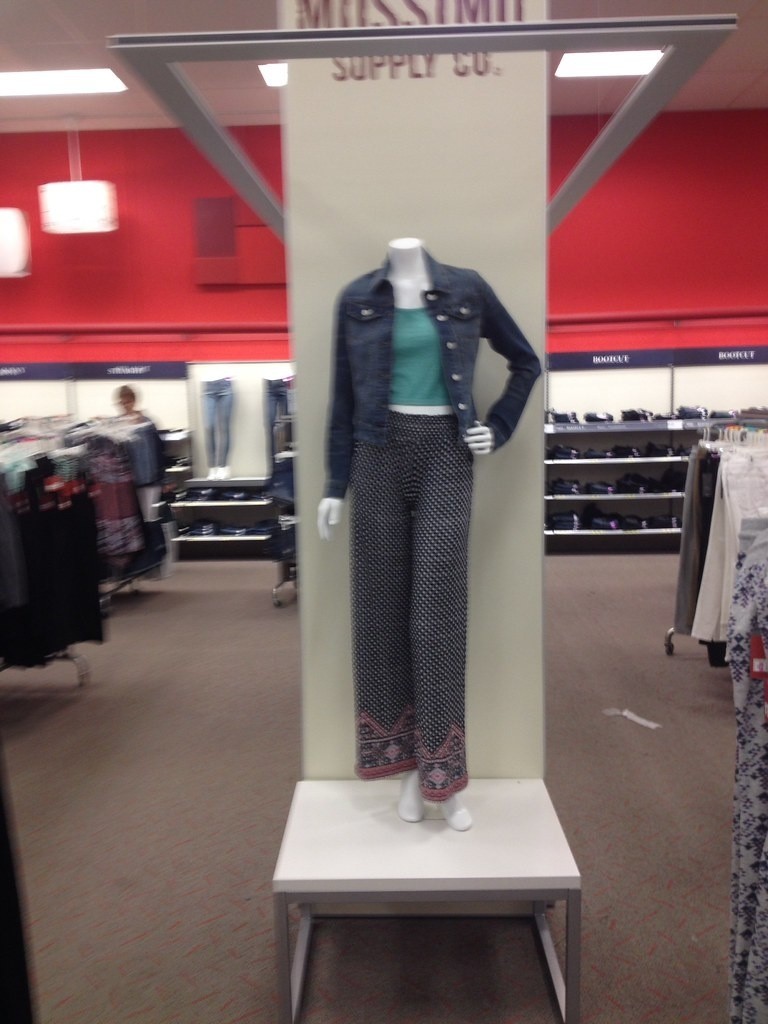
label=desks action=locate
[273,780,582,1024]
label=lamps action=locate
[0,116,118,280]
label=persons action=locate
[119,386,150,425]
[198,365,233,480]
[316,238,543,830]
[259,364,294,476]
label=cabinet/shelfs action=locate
[545,417,768,535]
[160,431,272,541]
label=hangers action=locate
[699,425,768,453]
